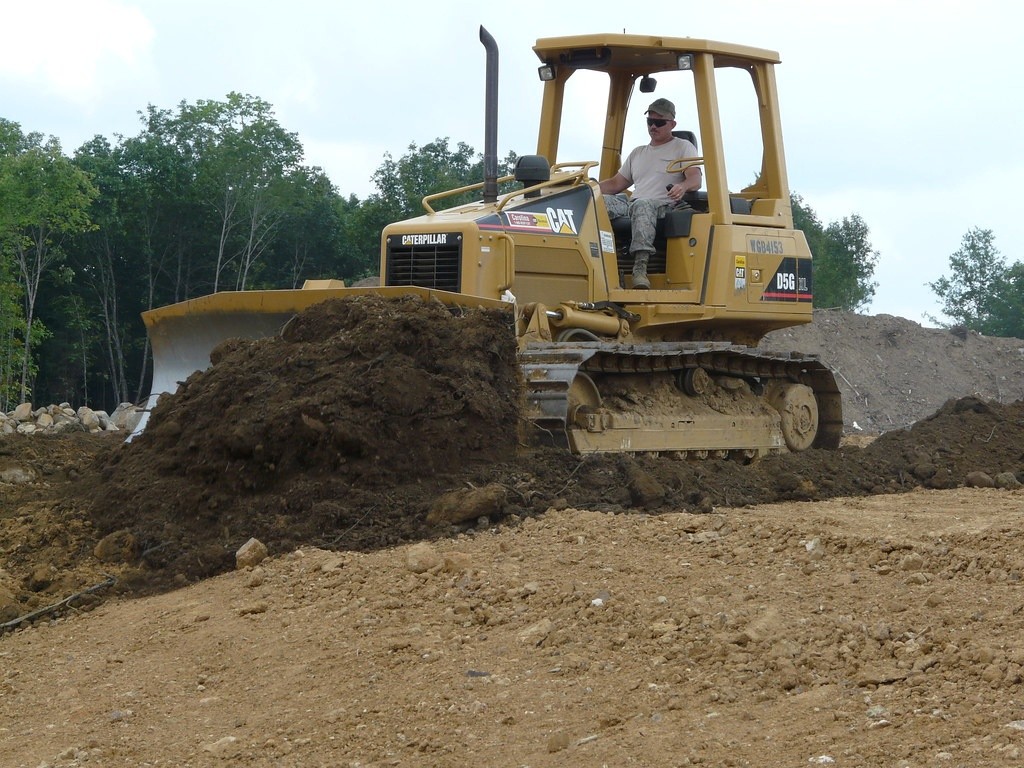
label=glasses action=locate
[645,117,672,128]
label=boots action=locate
[631,251,651,289]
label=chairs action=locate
[612,131,698,233]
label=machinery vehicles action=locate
[116,22,843,470]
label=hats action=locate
[643,98,676,118]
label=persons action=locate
[598,99,702,288]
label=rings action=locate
[678,194,679,196]
